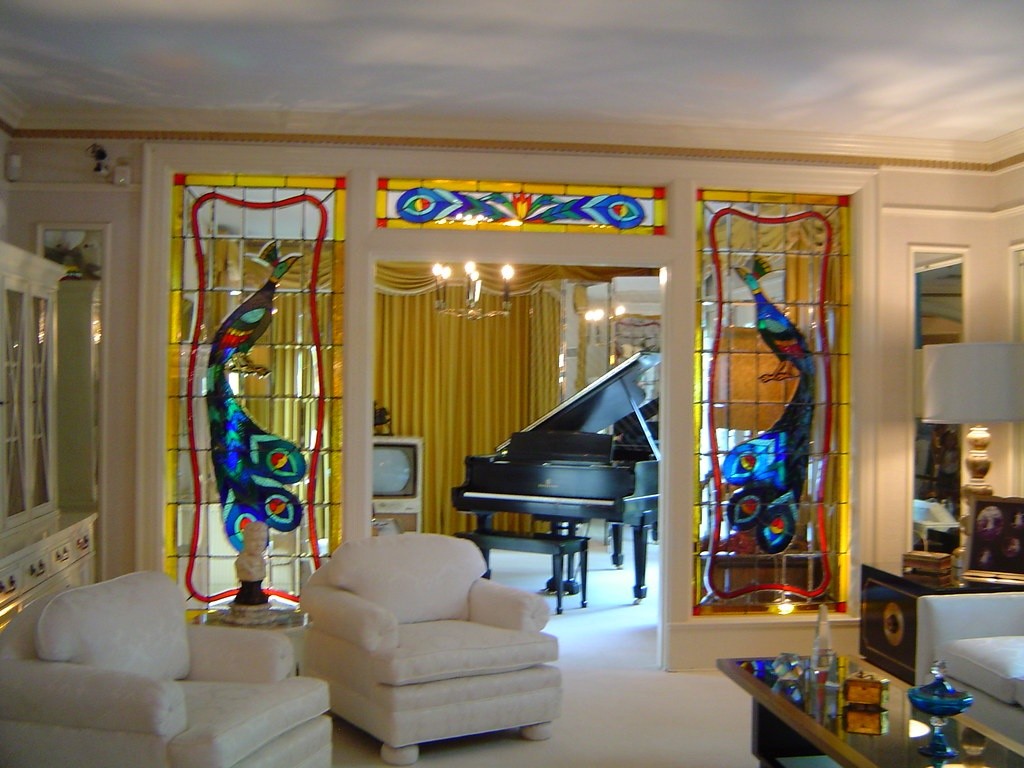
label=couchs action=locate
[916,591,1024,745]
[298,533,563,765]
[0,569,333,768]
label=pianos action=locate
[450,350,660,606]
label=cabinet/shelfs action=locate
[699,551,823,601]
[859,562,1024,686]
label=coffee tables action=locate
[717,650,1024,768]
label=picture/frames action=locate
[960,494,1024,580]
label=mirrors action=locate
[908,245,967,553]
[37,224,106,505]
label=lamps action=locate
[431,260,516,320]
[920,343,1024,568]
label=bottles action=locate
[810,604,833,671]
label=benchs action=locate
[457,528,591,613]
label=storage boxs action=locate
[903,550,952,574]
[844,672,890,706]
